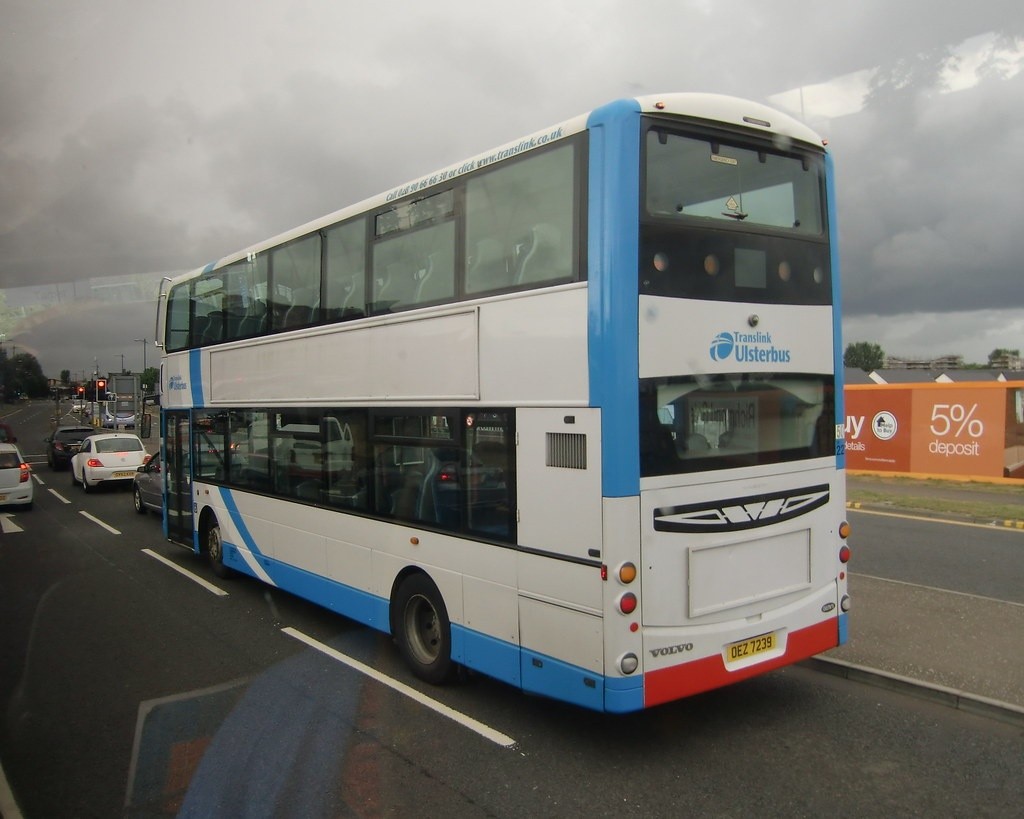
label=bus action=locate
[100,375,140,429]
[153,92,855,714]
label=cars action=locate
[43,425,97,473]
[278,417,354,484]
[0,442,34,511]
[353,443,508,527]
[132,450,191,518]
[72,400,87,413]
[70,433,152,494]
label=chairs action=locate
[375,260,416,313]
[200,311,224,345]
[339,272,364,317]
[235,300,264,339]
[467,236,508,292]
[283,287,312,328]
[309,299,320,323]
[717,430,738,448]
[515,223,562,285]
[682,433,711,453]
[415,248,454,302]
[183,315,208,348]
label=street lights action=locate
[112,354,123,373]
[133,338,147,370]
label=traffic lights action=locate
[96,380,118,403]
[78,387,85,398]
